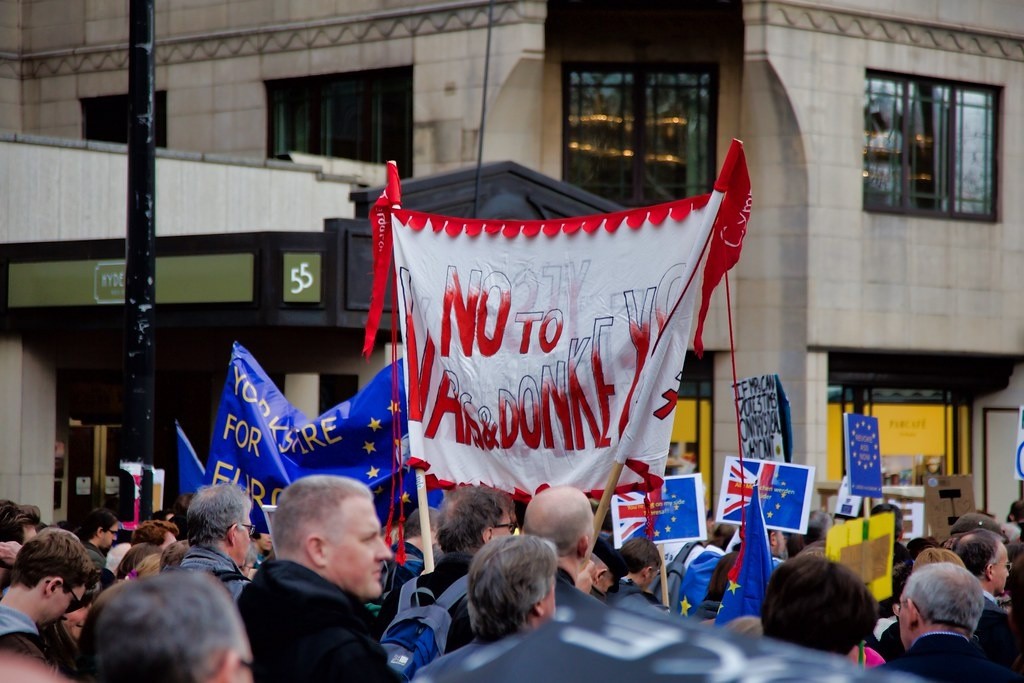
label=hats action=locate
[592,536,629,593]
[950,512,1009,545]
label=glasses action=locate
[109,529,118,535]
[893,600,920,617]
[984,563,1011,571]
[227,523,255,536]
[653,568,660,575]
[490,522,517,535]
[46,580,84,613]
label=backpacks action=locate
[380,573,469,682]
[380,553,423,600]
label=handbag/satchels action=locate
[652,541,704,613]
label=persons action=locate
[0,474,1023,683]
[235,475,412,683]
